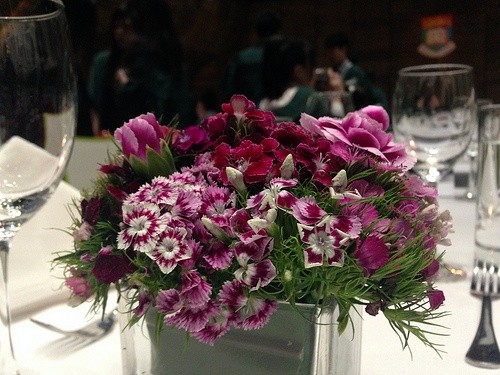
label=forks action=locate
[47,304,119,358]
[465,257,500,368]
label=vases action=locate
[146,301,362,375]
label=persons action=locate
[86,2,389,139]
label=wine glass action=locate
[453,73,492,202]
[0,0,79,375]
[392,64,476,282]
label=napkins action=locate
[0,189,81,326]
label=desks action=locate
[0,176,500,375]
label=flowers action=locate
[44,94,456,360]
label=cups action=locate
[472,104,500,249]
